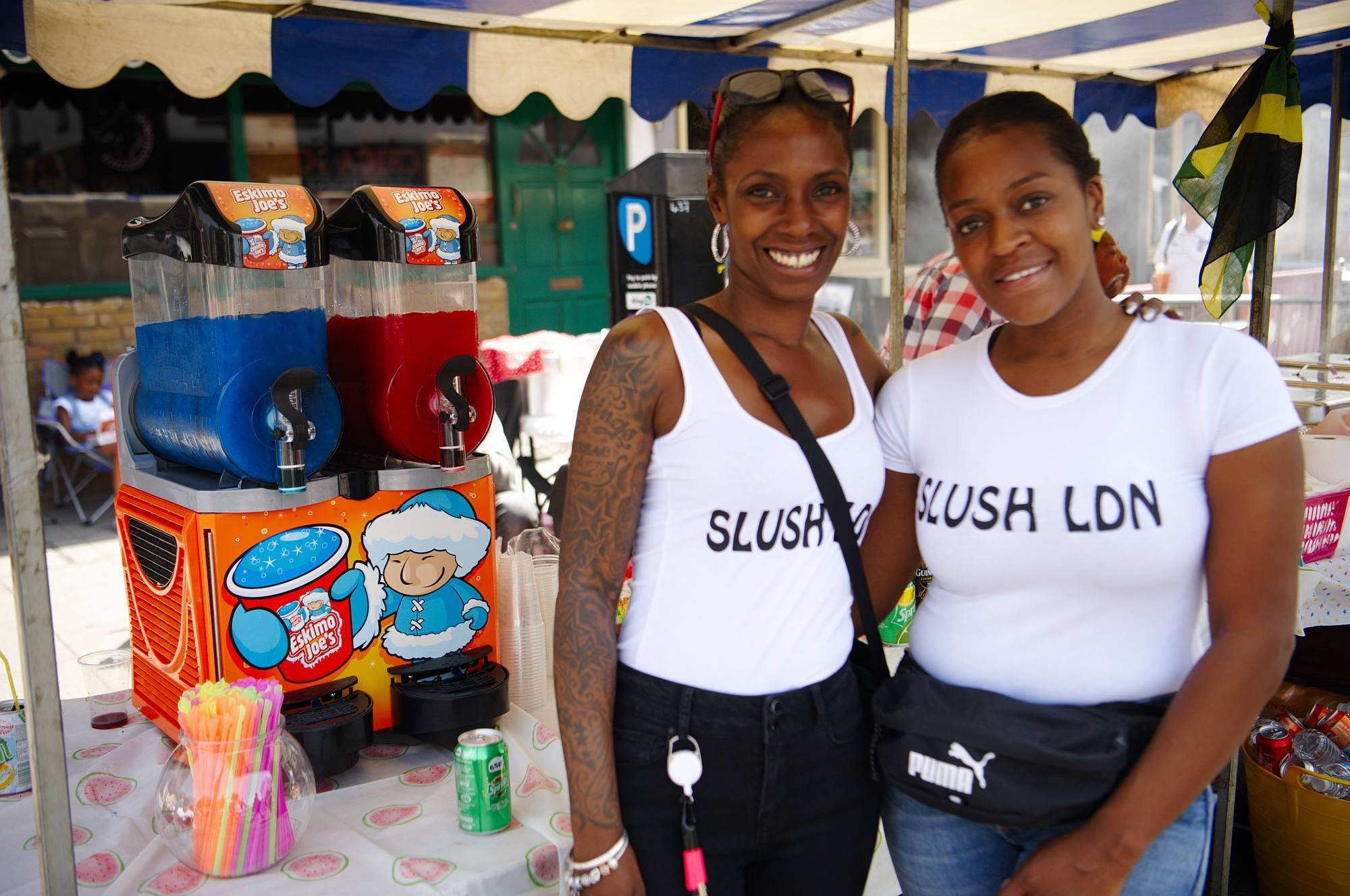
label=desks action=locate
[0,680,576,895]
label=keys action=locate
[680,797,708,895]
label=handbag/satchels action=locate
[856,639,889,811]
[876,650,1134,827]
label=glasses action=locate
[709,68,855,172]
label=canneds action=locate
[454,729,512,836]
[878,581,917,646]
[0,695,33,796]
[1245,702,1350,795]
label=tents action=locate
[1,1,1350,895]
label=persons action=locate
[1150,193,1249,336]
[51,347,116,461]
[852,91,1307,895]
[880,220,1128,615]
[551,71,1182,896]
[476,410,538,555]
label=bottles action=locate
[1292,729,1350,798]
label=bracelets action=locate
[564,830,629,889]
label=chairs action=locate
[36,358,119,525]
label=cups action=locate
[77,649,133,730]
